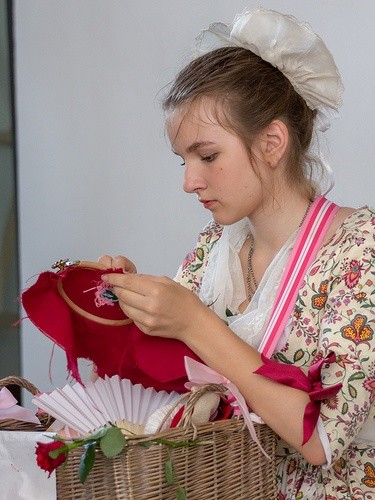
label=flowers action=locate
[35,423,213,500]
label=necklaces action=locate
[246,187,316,303]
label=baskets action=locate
[0,375,55,432]
[55,383,281,500]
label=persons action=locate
[97,7,375,500]
